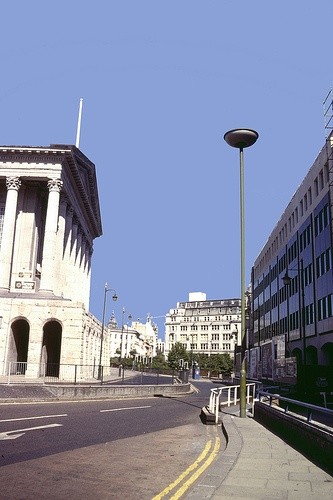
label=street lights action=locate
[280,257,308,403]
[97,281,118,381]
[118,306,133,377]
[244,306,260,346]
